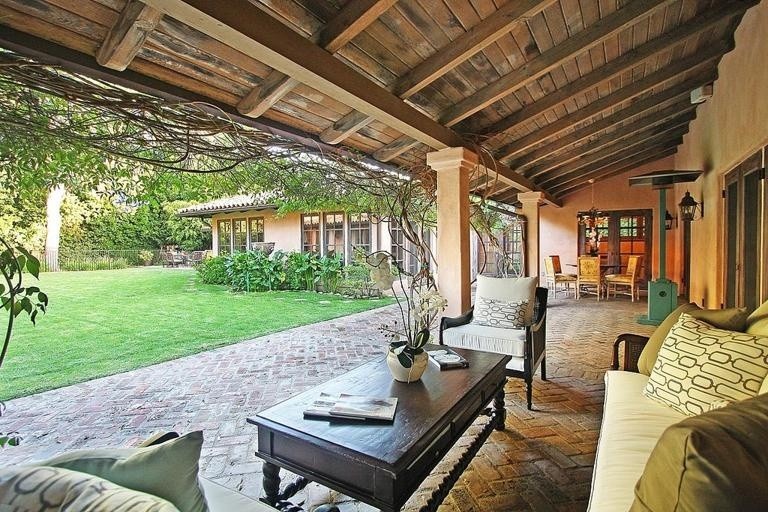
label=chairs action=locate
[0,430,282,512]
[544,255,644,302]
[438,286,548,408]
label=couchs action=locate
[584,333,689,512]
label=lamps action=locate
[576,178,612,225]
[665,210,677,230]
[678,189,704,222]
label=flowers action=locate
[377,288,449,369]
[589,227,602,250]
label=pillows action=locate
[0,465,183,512]
[471,294,531,330]
[642,313,768,417]
[473,274,539,326]
[637,301,748,375]
[629,392,767,510]
[746,297,767,397]
[27,430,212,512]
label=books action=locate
[329,394,399,420]
[303,393,366,420]
[427,348,468,369]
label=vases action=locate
[590,249,598,257]
[387,347,429,383]
